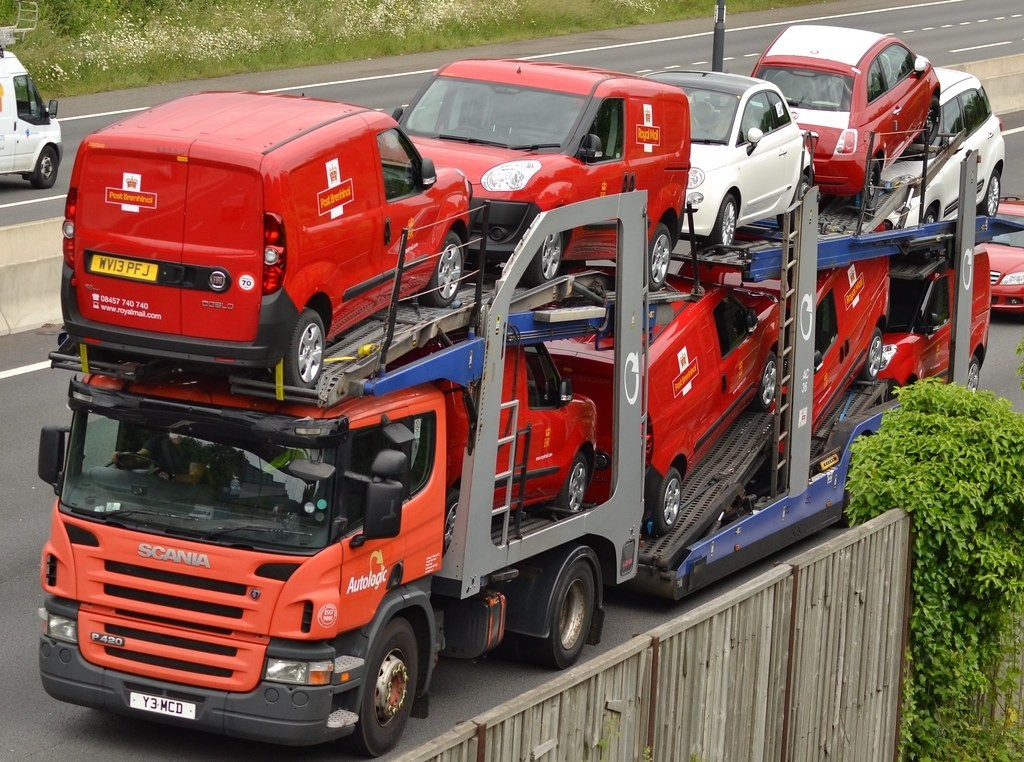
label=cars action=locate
[881,66,1005,233]
[639,69,816,252]
[754,23,941,208]
[989,197,1024,310]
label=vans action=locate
[387,338,601,555]
[380,58,692,288]
[879,244,990,410]
[678,222,891,467]
[0,50,65,187]
[59,89,470,387]
[525,283,781,535]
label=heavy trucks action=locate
[33,213,993,761]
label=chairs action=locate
[692,100,714,131]
